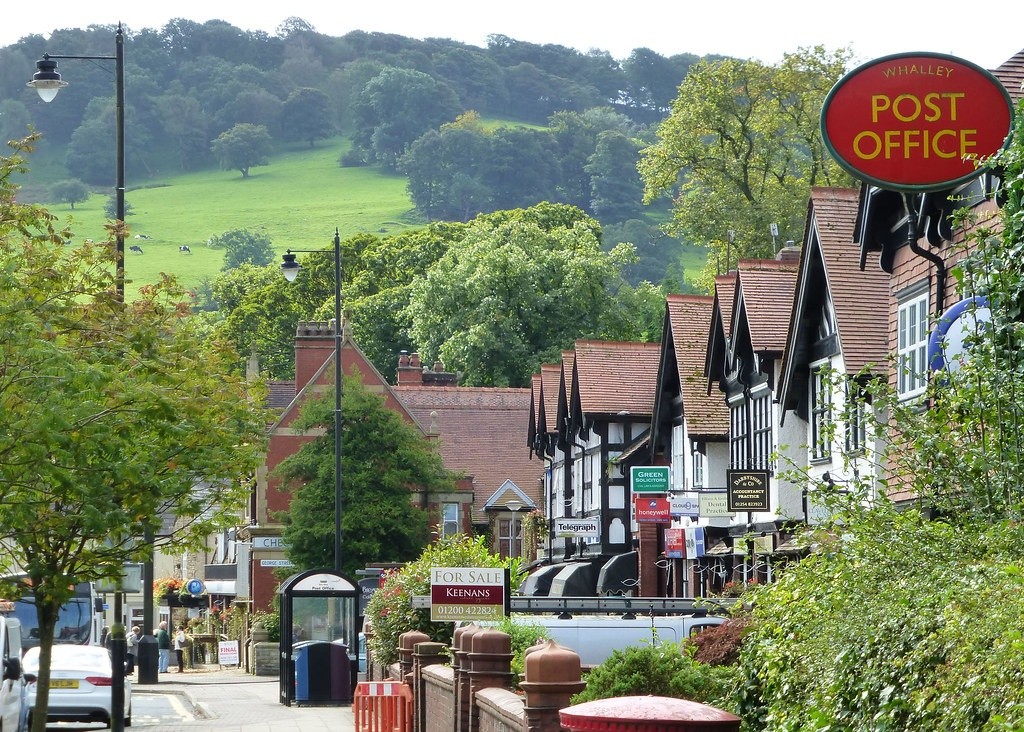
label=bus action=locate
[0,570,102,660]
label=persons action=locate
[175,626,185,673]
[293,624,307,643]
[157,621,173,673]
[100,625,139,677]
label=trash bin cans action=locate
[294,643,352,708]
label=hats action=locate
[177,626,183,629]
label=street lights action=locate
[26,19,127,732]
[280,226,343,641]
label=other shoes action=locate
[159,671,170,673]
[175,671,184,674]
[127,672,133,676]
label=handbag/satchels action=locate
[126,633,136,647]
[178,639,189,648]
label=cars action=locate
[22,644,133,727]
